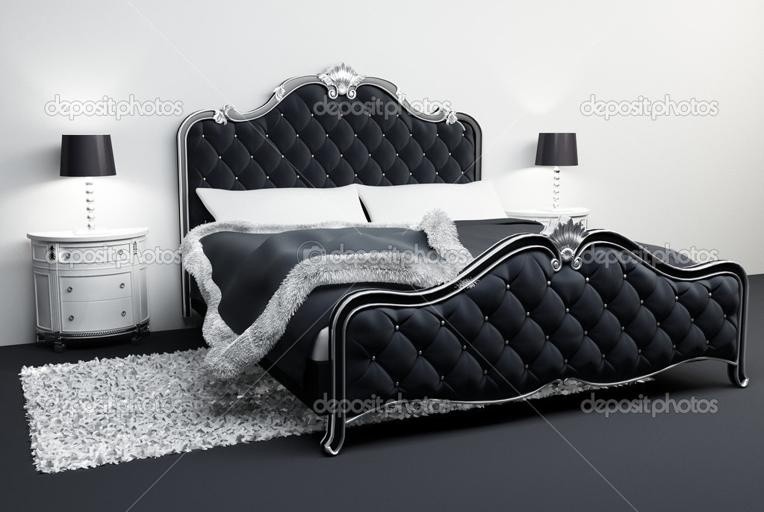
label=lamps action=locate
[59,133,117,233]
[535,132,580,213]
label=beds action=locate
[177,59,751,454]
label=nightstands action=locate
[22,232,154,346]
[506,211,588,236]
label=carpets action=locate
[18,347,326,473]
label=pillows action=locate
[195,180,509,223]
